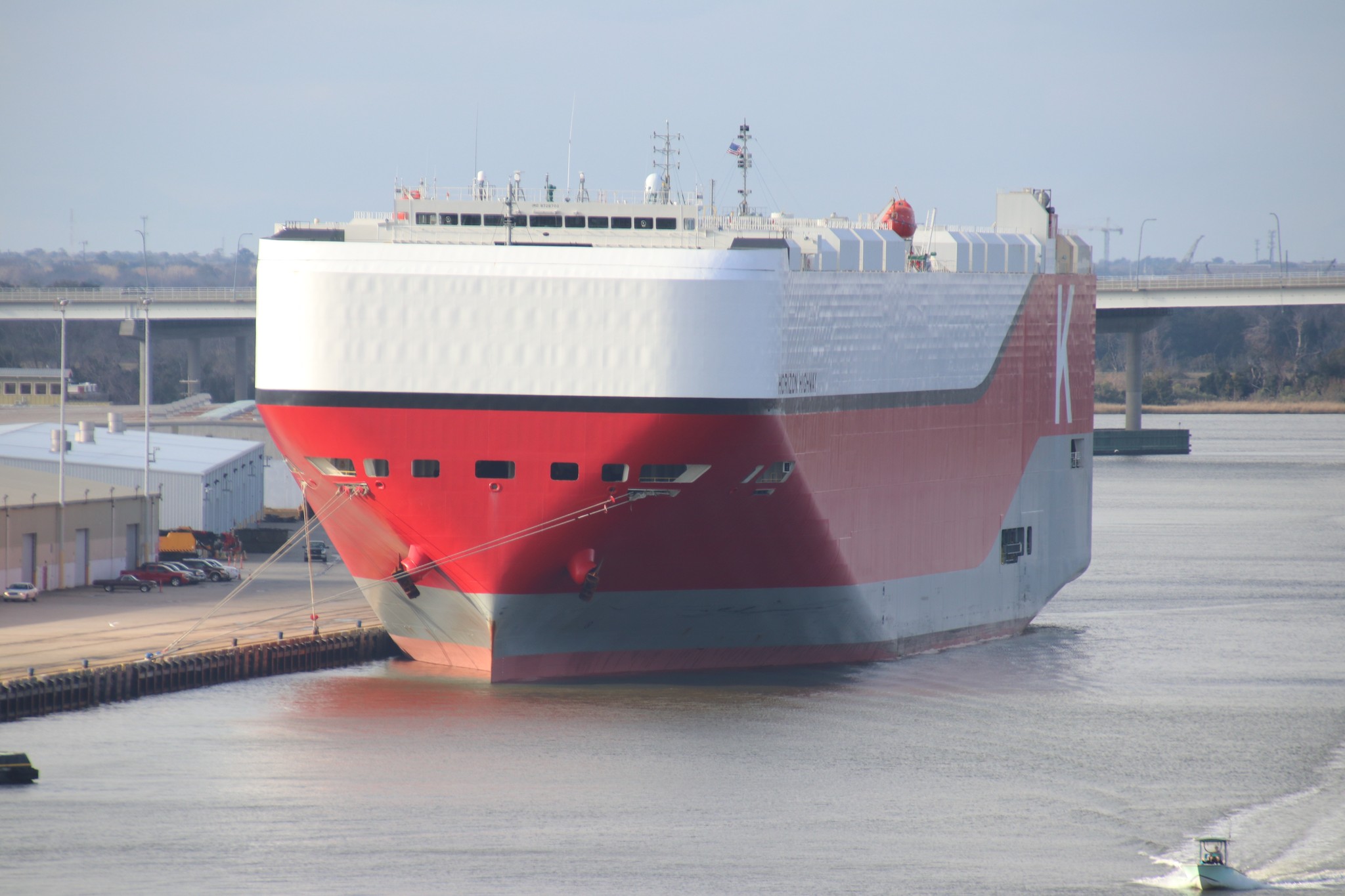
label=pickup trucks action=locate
[92,574,157,593]
[141,558,238,585]
[118,564,192,587]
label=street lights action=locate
[58,296,69,594]
[1136,217,1157,290]
[142,297,153,565]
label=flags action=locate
[726,141,742,156]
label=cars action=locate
[2,582,39,602]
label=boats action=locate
[250,116,1098,685]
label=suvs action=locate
[302,540,330,562]
